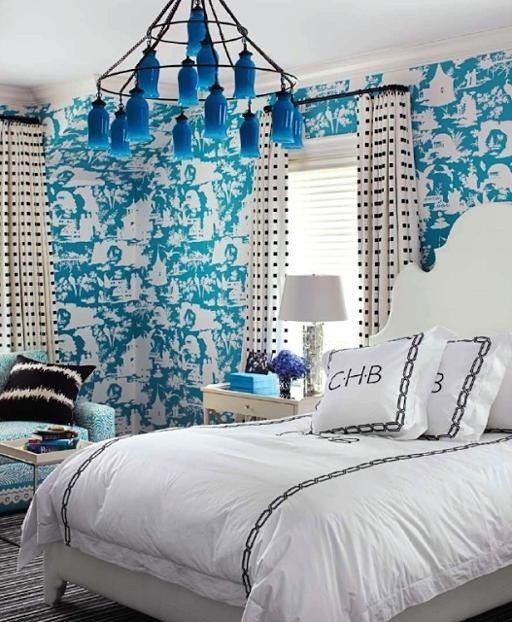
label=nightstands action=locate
[200,380,324,429]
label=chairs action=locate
[0,350,115,522]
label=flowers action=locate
[267,349,308,397]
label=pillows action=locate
[309,330,441,439]
[0,354,97,426]
[421,330,504,447]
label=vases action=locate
[278,377,291,397]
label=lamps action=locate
[85,0,304,161]
[278,273,348,397]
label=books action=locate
[23,426,81,454]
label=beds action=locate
[20,200,512,619]
